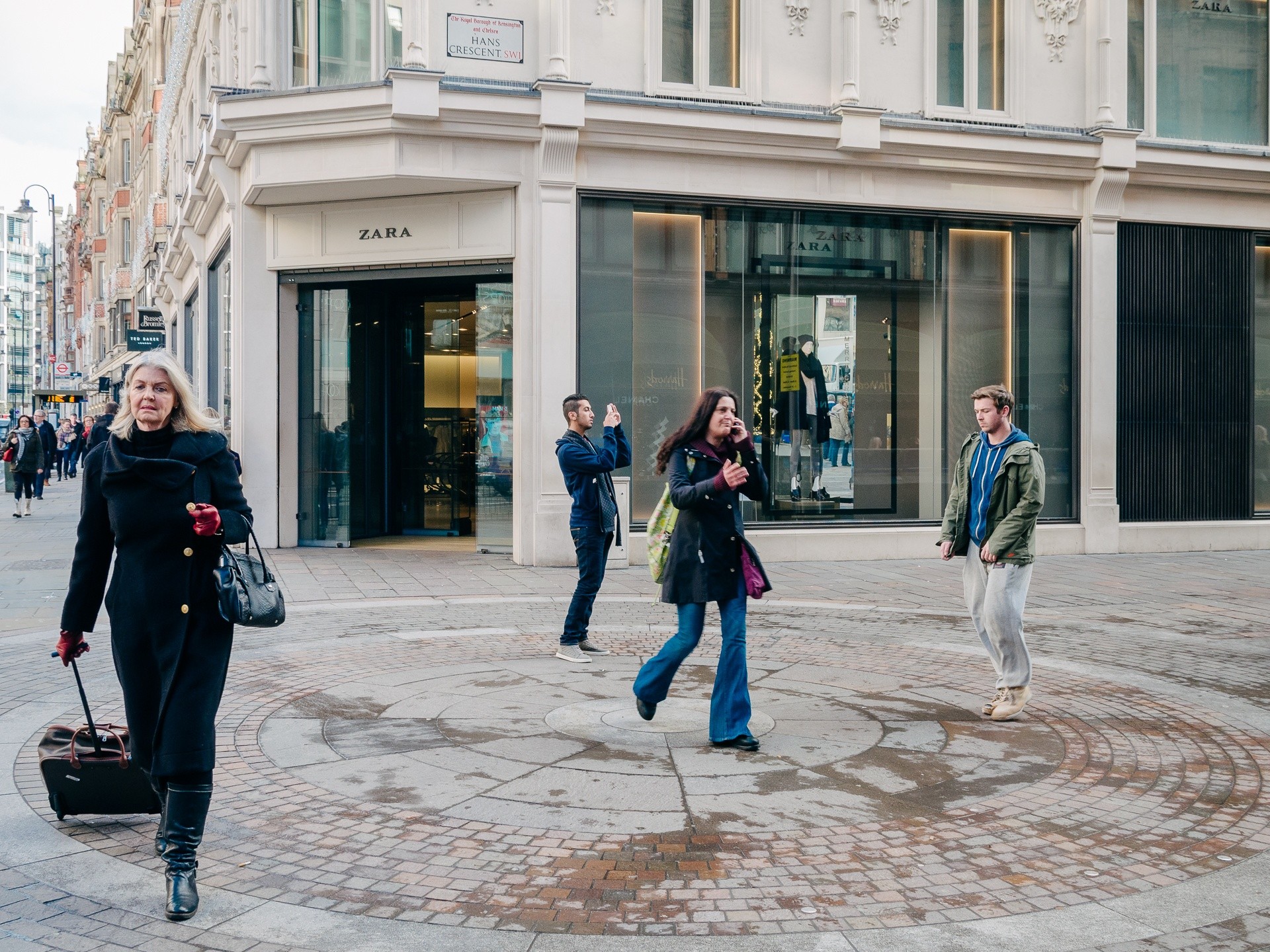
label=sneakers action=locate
[983,686,1032,720]
[557,638,610,663]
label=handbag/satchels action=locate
[2,446,13,462]
[36,723,162,821]
[647,456,695,585]
[217,514,287,627]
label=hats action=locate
[798,334,815,347]
[781,336,796,351]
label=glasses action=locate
[19,420,29,423]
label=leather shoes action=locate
[789,489,800,502]
[636,697,657,721]
[712,736,761,750]
[820,487,830,498]
[809,489,825,501]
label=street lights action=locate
[13,184,57,391]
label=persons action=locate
[555,394,631,663]
[632,388,773,750]
[935,385,1046,721]
[479,409,510,468]
[789,334,832,502]
[774,336,830,498]
[55,350,254,921]
[2,402,120,518]
[298,411,349,541]
[823,394,855,467]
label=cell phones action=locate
[731,423,739,436]
[606,405,613,413]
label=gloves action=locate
[57,629,90,667]
[189,503,221,536]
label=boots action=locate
[24,498,32,516]
[143,757,167,855]
[163,782,213,919]
[12,498,22,518]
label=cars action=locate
[0,418,18,442]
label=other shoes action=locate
[842,463,851,467]
[831,465,838,468]
[57,474,67,481]
[32,490,43,500]
[44,479,50,485]
